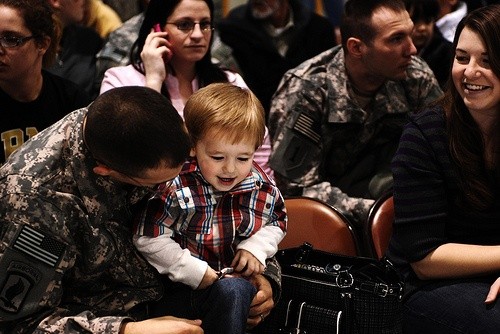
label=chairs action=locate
[279,192,395,260]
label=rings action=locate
[260,315,264,321]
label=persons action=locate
[0,0,274,184]
[405,0,468,93]
[394,0,500,334]
[219,0,446,258]
[0,83,288,334]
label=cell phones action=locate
[153,24,171,63]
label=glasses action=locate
[0,33,34,49]
[165,21,216,32]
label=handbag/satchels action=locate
[261,243,403,334]
